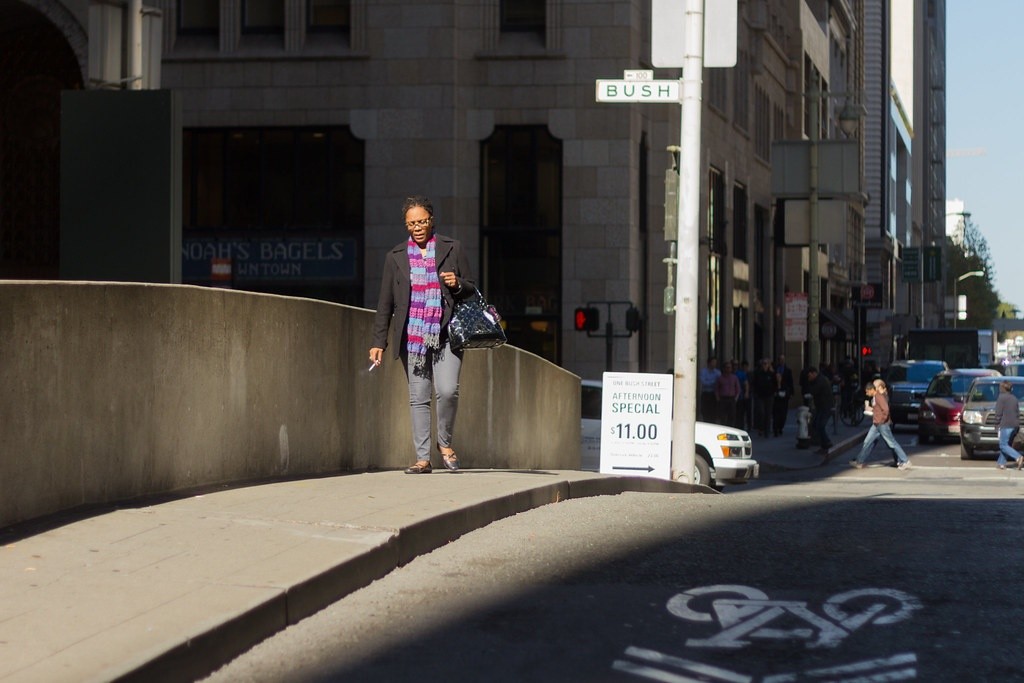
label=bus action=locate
[908,329,993,368]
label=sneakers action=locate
[995,463,1007,470]
[848,460,863,470]
[897,459,912,472]
[1015,455,1024,471]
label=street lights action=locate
[918,213,970,327]
[952,271,985,328]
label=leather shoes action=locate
[404,461,433,475]
[435,440,461,472]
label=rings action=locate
[448,281,451,285]
[369,356,372,359]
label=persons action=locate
[369,195,476,474]
[730,359,750,429]
[754,358,781,439]
[740,360,751,371]
[773,355,794,435]
[873,379,902,467]
[994,380,1023,469]
[807,366,835,455]
[715,362,741,427]
[699,357,721,422]
[848,381,912,470]
[801,355,873,405]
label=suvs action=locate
[886,360,1024,460]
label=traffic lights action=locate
[627,308,640,333]
[574,308,599,331]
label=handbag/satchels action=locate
[446,285,508,353]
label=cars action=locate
[581,378,758,492]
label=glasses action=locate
[405,215,431,230]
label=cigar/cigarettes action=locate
[369,360,378,372]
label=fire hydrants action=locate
[796,406,812,448]
